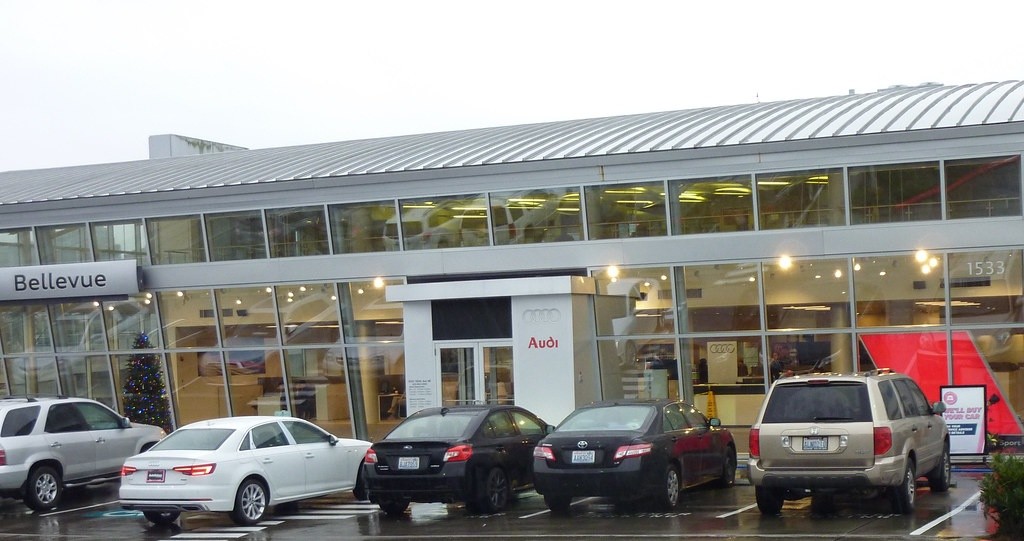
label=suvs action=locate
[746,367,952,518]
[916,248,1023,371]
[230,210,321,259]
[458,189,562,245]
[383,198,469,250]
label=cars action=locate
[605,277,668,369]
[10,299,150,384]
[320,297,405,378]
[198,290,335,387]
[361,404,557,515]
[119,414,374,527]
[533,399,737,514]
[0,396,165,514]
[456,363,513,407]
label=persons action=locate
[387,390,407,420]
[646,347,789,392]
